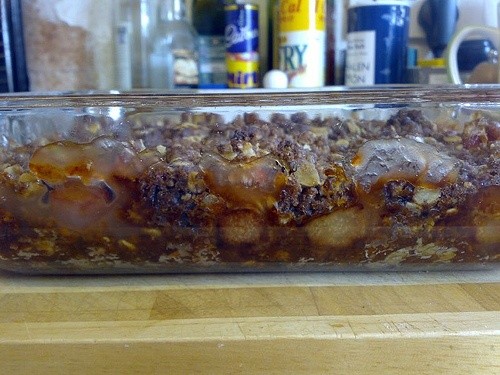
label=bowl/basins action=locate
[0,87,500,275]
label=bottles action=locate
[154,0,199,88]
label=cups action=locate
[113,0,154,86]
